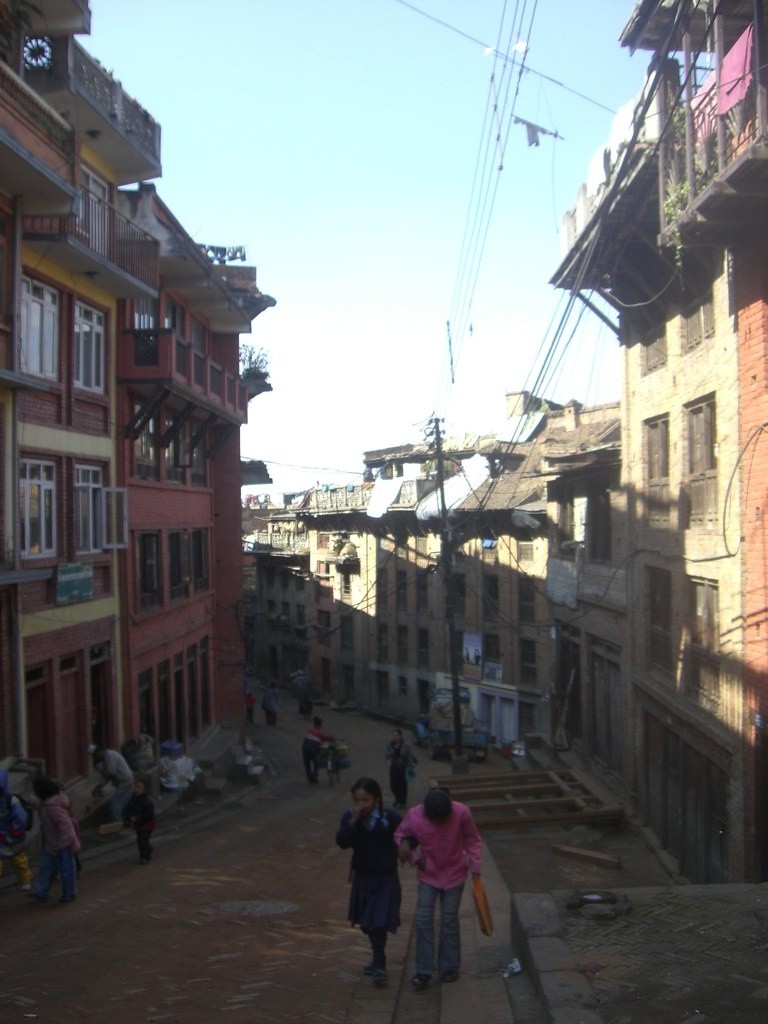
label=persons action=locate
[92,748,135,823]
[334,777,419,983]
[301,716,335,784]
[394,787,482,989]
[126,777,156,865]
[245,681,314,726]
[385,729,413,809]
[0,770,82,903]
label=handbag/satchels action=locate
[404,767,417,784]
[472,878,493,935]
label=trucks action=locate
[413,687,495,762]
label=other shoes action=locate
[411,973,432,988]
[22,884,31,892]
[373,965,390,983]
[58,895,78,903]
[441,970,458,981]
[25,892,45,899]
[364,960,374,971]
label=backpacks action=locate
[7,794,34,832]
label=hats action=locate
[313,716,322,726]
[87,744,97,754]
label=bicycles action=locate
[324,739,345,788]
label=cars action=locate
[288,671,313,697]
